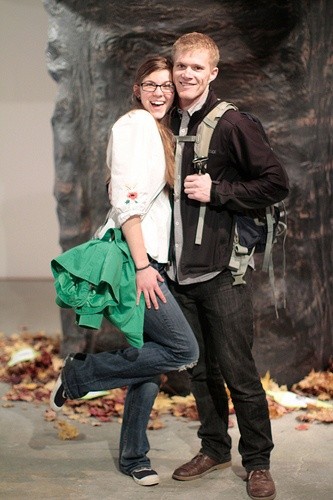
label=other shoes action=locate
[49,353,74,411]
[130,467,160,486]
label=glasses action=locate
[141,81,176,93]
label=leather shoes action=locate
[173,452,232,480]
[247,469,277,500]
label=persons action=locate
[164,32,291,500]
[49,53,200,488]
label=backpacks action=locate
[175,100,288,320]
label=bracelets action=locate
[133,262,152,271]
